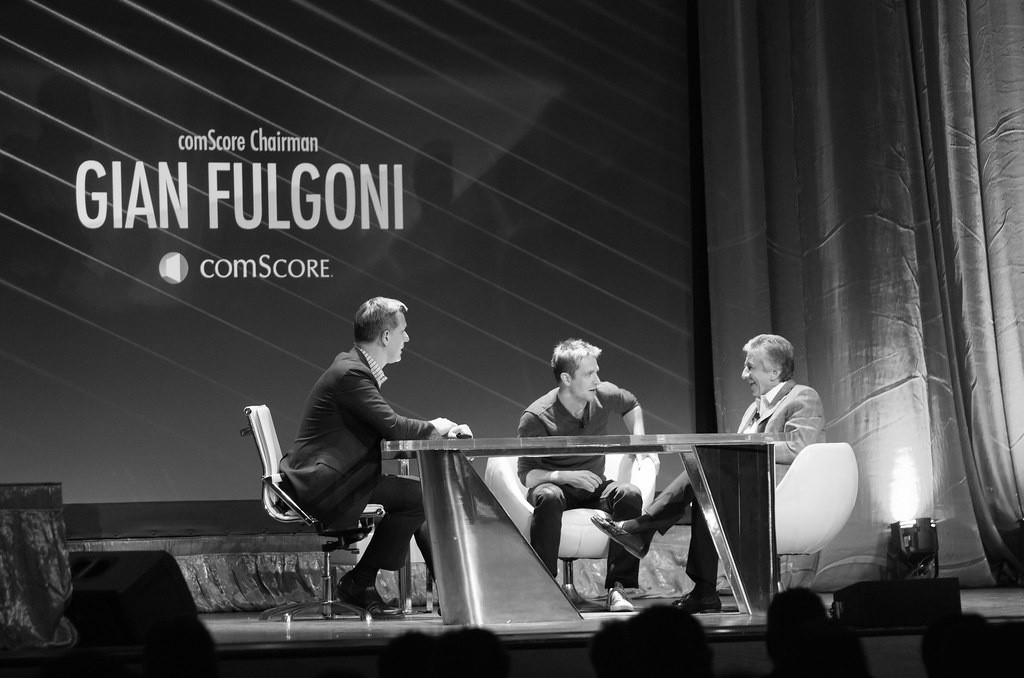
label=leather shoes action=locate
[591,514,649,559]
[335,570,405,619]
[671,590,722,614]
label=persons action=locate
[379,587,1024,678]
[277,297,473,618]
[33,616,220,678]
[590,334,826,615]
[517,339,661,611]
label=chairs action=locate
[239,401,390,630]
[482,454,660,616]
[771,441,863,597]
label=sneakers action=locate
[607,581,633,611]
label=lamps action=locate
[877,512,952,579]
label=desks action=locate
[380,430,783,635]
